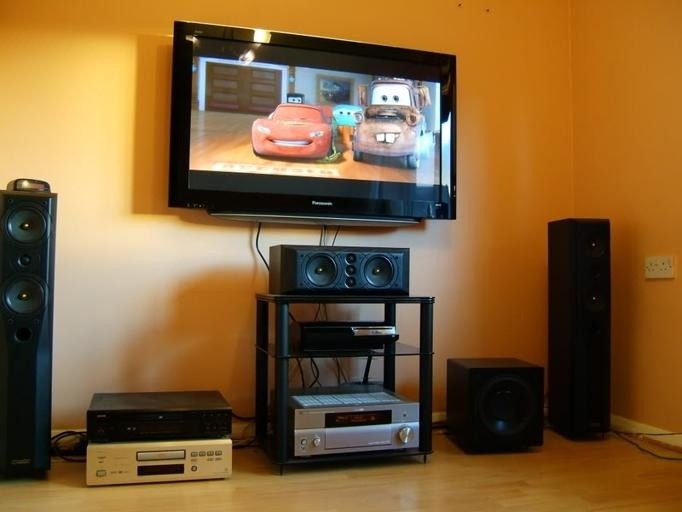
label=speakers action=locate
[0,190,58,477]
[448,359,543,454]
[548,219,611,440]
[270,245,409,295]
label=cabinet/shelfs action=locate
[256,293,436,474]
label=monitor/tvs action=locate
[168,20,457,227]
